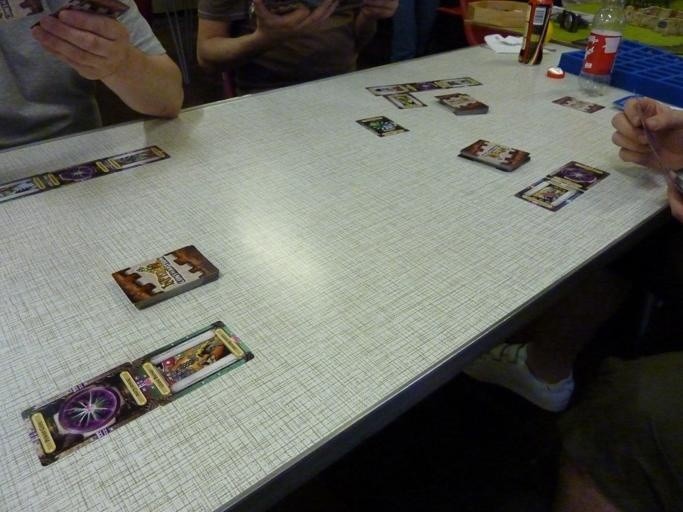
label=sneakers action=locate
[460,343,575,413]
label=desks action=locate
[0,38,683,512]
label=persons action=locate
[193,1,401,92]
[0,1,183,151]
[459,95,681,511]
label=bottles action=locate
[579,0,627,98]
[518,0,553,64]
